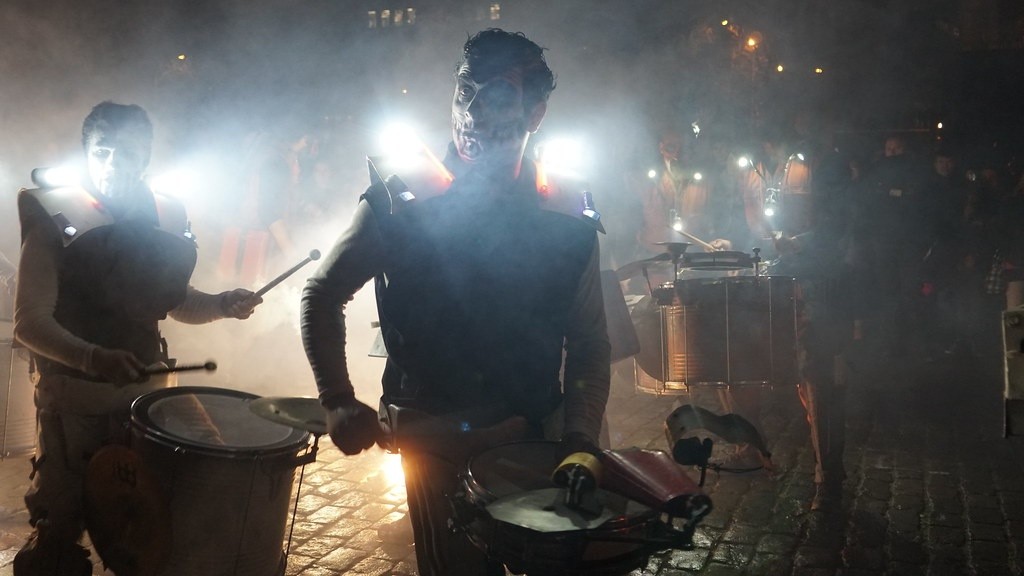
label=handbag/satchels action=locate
[986,246,1007,295]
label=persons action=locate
[631,116,1024,531]
[13,101,263,576]
[220,136,298,277]
[302,27,611,576]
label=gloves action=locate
[324,392,386,455]
[551,451,603,518]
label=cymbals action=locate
[249,396,329,434]
[615,241,698,280]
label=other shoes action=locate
[812,480,843,514]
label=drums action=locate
[129,384,312,576]
[443,249,799,575]
